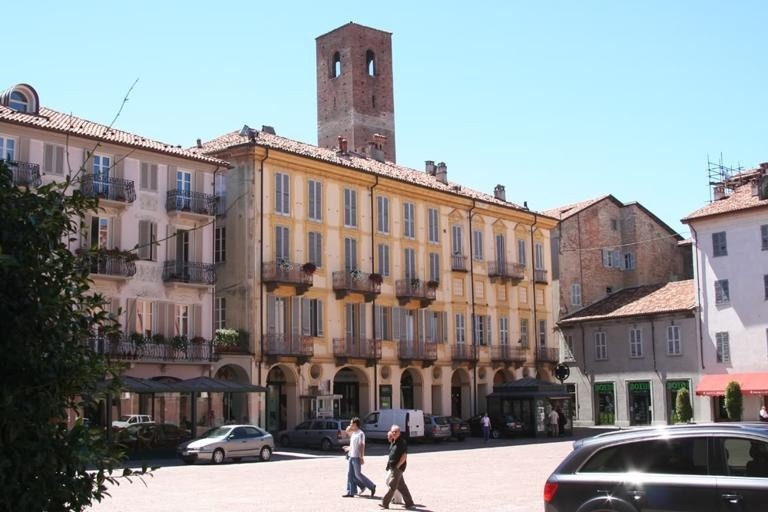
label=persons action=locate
[759,405,768,421]
[547,407,559,438]
[342,417,376,498]
[386,431,403,504]
[480,412,492,444]
[377,424,416,510]
[341,424,366,495]
[556,409,565,437]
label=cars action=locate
[425,413,526,442]
[112,415,367,464]
[544,421,768,512]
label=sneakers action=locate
[340,484,417,511]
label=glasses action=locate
[390,430,398,434]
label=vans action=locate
[363,409,424,445]
